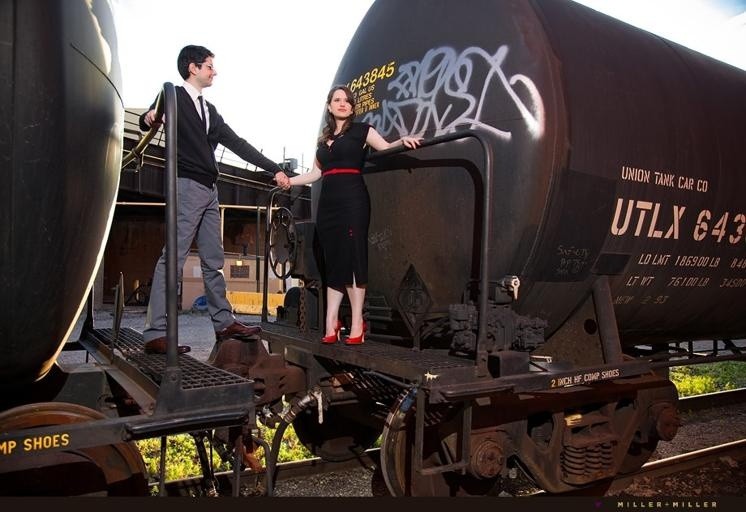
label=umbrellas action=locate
[144,337,190,354]
[216,322,261,340]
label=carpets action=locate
[198,96,206,132]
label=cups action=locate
[196,63,212,69]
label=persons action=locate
[273,86,425,345]
[139,45,290,355]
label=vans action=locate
[322,320,342,343]
[345,322,367,344]
